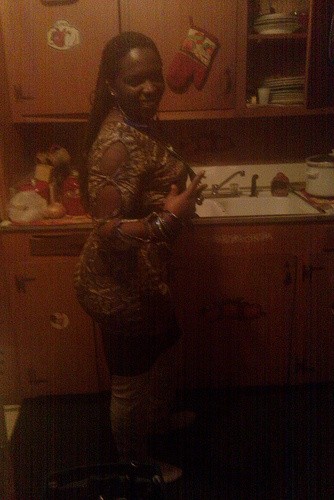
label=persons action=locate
[74,32,208,483]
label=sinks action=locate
[218,195,321,217]
[194,196,227,218]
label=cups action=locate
[18,181,49,204]
[258,88,270,104]
[62,177,85,216]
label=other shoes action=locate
[152,407,197,433]
[118,456,182,485]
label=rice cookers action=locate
[305,153,334,197]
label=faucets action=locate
[211,170,245,196]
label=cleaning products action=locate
[270,172,289,196]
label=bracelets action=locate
[144,211,178,239]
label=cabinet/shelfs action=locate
[184,236,334,387]
[0,0,247,114]
[248,0,334,117]
[0,234,114,398]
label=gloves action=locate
[166,25,219,93]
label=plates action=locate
[264,77,305,104]
[252,14,301,35]
